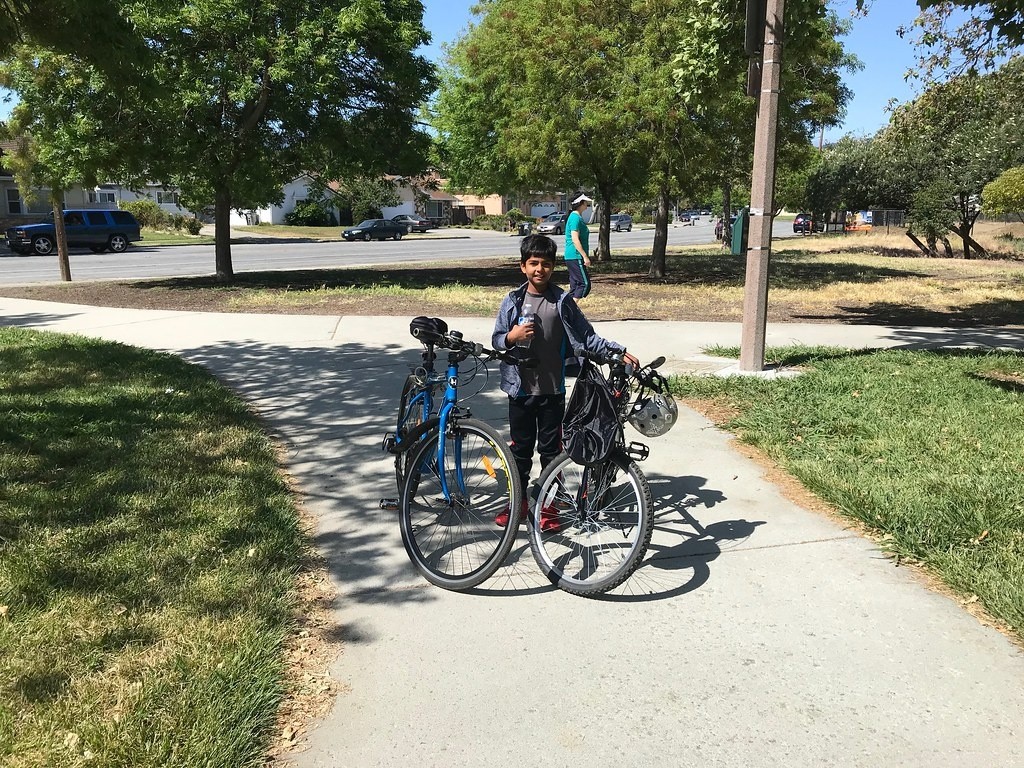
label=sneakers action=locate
[495,501,528,526]
[539,499,561,532]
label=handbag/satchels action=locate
[561,358,619,465]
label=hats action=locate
[571,193,592,205]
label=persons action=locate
[564,193,593,321]
[492,235,640,533]
[419,207,425,218]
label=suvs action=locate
[5,208,143,255]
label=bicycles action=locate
[526,352,677,598]
[379,317,522,593]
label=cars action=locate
[535,214,565,236]
[390,215,431,234]
[541,212,566,220]
[341,219,408,242]
[608,214,633,232]
[793,214,825,233]
[680,210,709,223]
[716,218,738,240]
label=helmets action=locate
[628,393,678,438]
